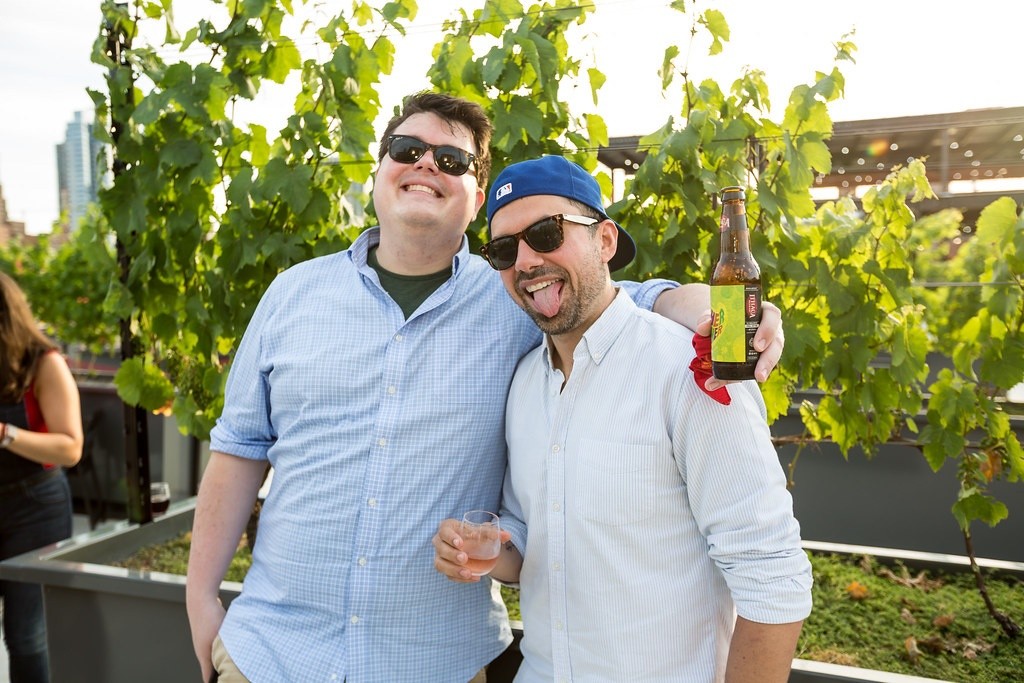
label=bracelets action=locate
[0,423,18,448]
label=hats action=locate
[486,153,632,273]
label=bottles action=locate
[710,186,761,381]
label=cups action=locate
[150,482,170,515]
[459,510,501,576]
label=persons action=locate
[185,93,814,683]
[0,270,84,683]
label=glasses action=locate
[480,212,598,270]
[379,134,481,177]
